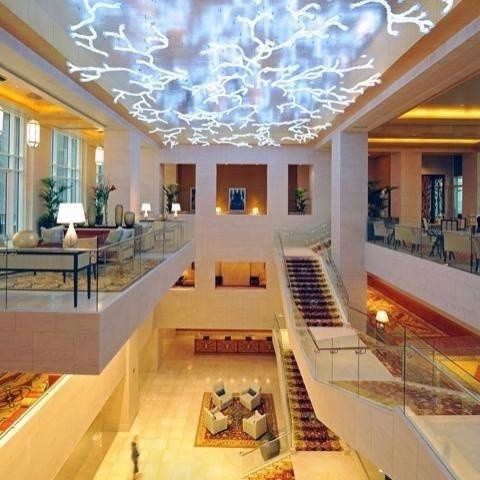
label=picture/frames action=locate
[228,187,247,213]
[189,186,195,211]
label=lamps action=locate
[57,202,86,246]
[171,202,181,220]
[26,92,41,151]
[141,202,152,220]
[0,104,4,136]
[374,310,389,331]
[94,128,104,168]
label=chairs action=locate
[202,381,267,439]
[382,215,479,274]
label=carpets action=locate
[144,238,191,253]
[194,393,279,448]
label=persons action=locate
[130,433,142,480]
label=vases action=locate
[124,211,135,228]
[114,204,123,227]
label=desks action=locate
[0,219,185,308]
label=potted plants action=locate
[368,179,399,241]
[88,183,116,224]
[37,178,68,244]
